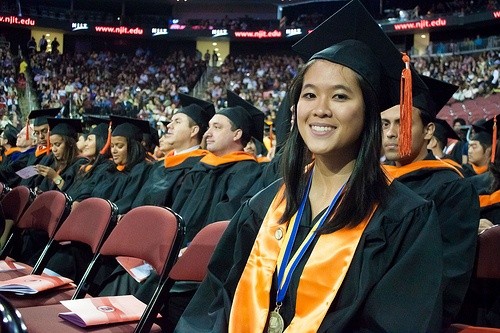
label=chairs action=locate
[436,94,500,128]
[0,184,231,333]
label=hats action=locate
[160,121,171,135]
[99,115,151,154]
[291,0,429,158]
[86,116,111,129]
[429,118,462,142]
[215,88,265,143]
[47,117,83,155]
[3,123,19,144]
[26,107,62,140]
[471,114,500,163]
[399,73,459,116]
[173,92,215,133]
[149,126,160,148]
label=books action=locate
[0,261,26,271]
[0,275,77,295]
[115,256,155,283]
[58,295,162,327]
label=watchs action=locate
[55,177,63,184]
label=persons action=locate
[0,0,500,327]
[173,0,444,333]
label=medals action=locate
[263,300,284,333]
